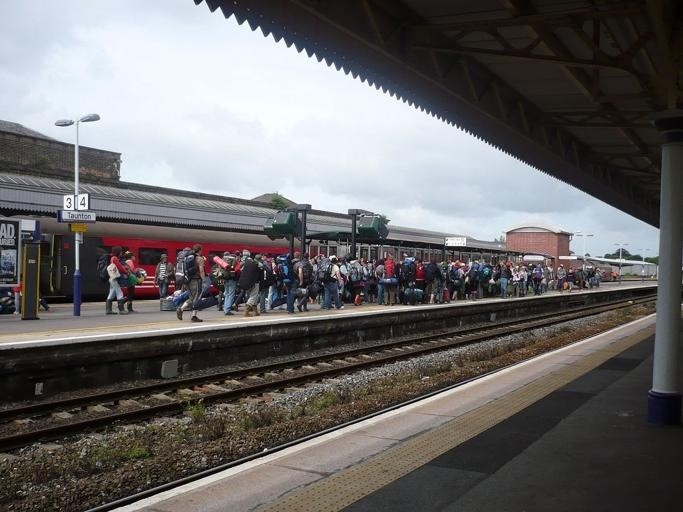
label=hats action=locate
[161,254,168,258]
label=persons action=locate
[490,260,617,299]
[106,247,140,315]
[13,274,23,315]
[154,245,495,322]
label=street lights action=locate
[639,248,649,283]
[54,114,100,316]
[615,243,628,285]
[575,233,593,272]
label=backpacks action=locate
[379,260,541,301]
[96,253,120,282]
[175,250,199,285]
[210,253,374,290]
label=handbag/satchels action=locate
[115,268,147,287]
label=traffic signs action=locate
[57,210,96,224]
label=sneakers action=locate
[191,316,202,322]
[217,298,344,316]
[177,307,183,320]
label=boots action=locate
[106,297,135,314]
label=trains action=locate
[0,172,557,299]
